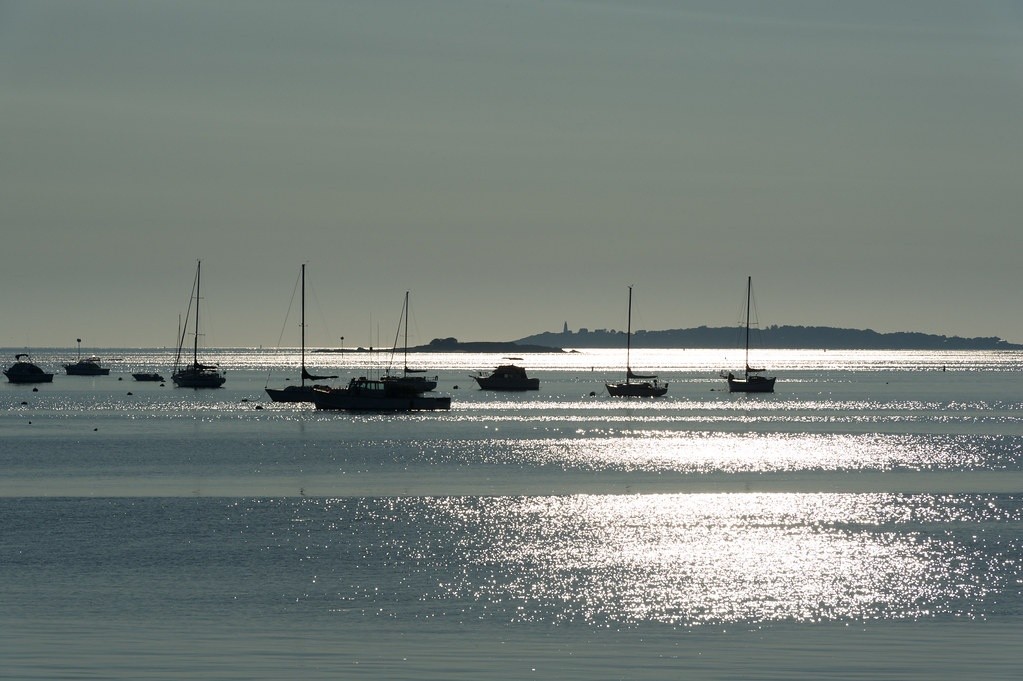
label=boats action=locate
[468,362,540,391]
[2,353,54,384]
[63,356,110,375]
[132,372,164,381]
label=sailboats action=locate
[170,259,227,388]
[266,263,452,412]
[605,283,670,398]
[711,276,777,393]
